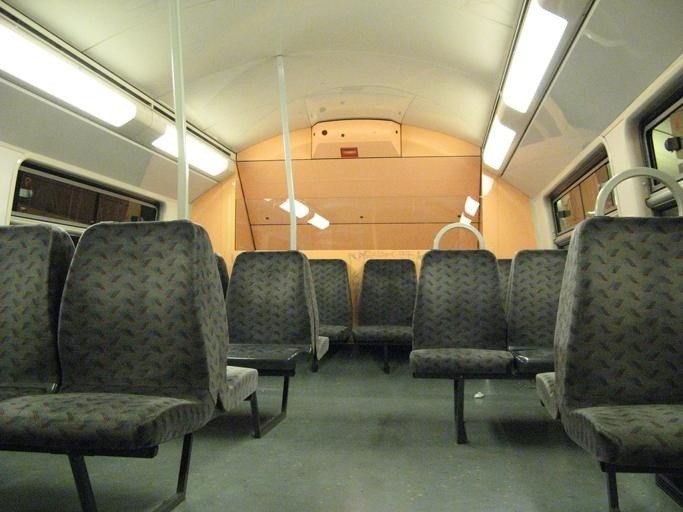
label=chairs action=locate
[0,219,353,510]
[354,217,682,511]
